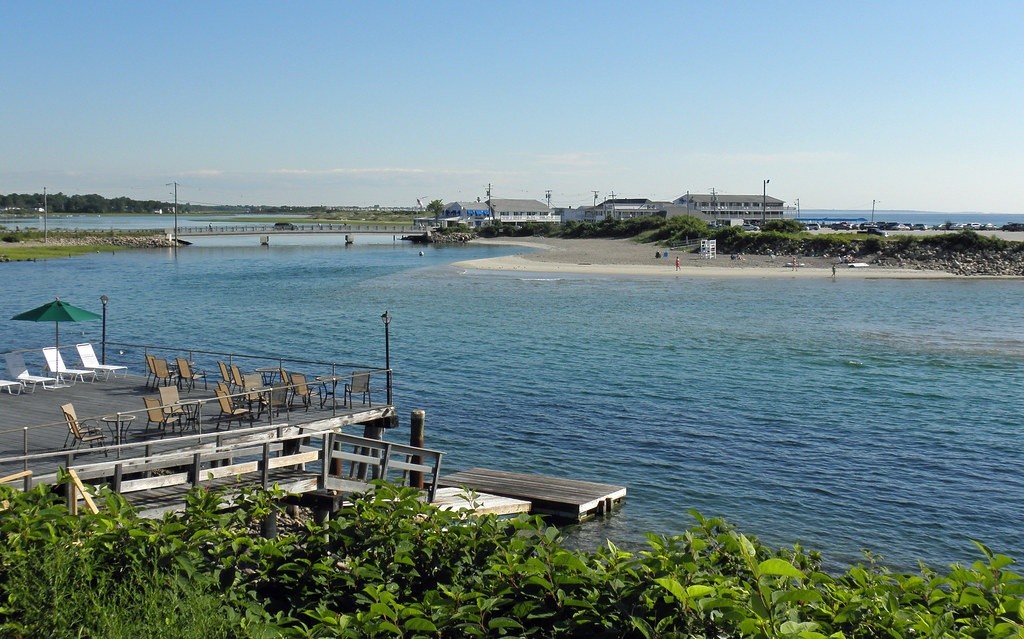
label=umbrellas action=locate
[11,297,102,386]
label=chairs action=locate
[0,343,371,461]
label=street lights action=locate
[380,309,393,404]
[99,294,108,364]
[763,180,770,229]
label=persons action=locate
[209,223,212,232]
[420,223,424,228]
[831,265,835,278]
[676,257,680,271]
[791,258,797,271]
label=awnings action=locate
[446,210,460,214]
[467,210,489,214]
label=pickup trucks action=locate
[830,221,849,229]
[857,228,886,238]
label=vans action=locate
[803,224,821,230]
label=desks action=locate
[251,386,276,420]
[101,415,136,454]
[316,375,343,410]
[168,361,194,391]
[256,368,280,388]
[175,399,206,435]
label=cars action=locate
[848,221,994,231]
[742,224,761,231]
[273,222,299,231]
